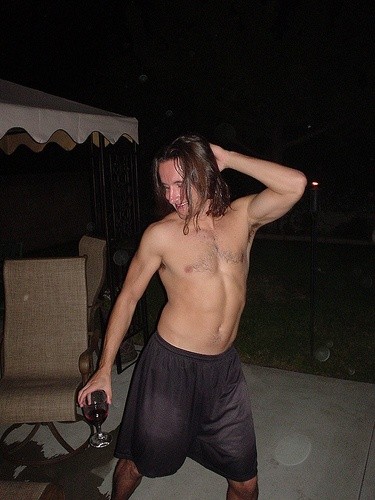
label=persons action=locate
[77,133,308,500]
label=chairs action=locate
[78,234,126,378]
[0,257,110,466]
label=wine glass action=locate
[82,390,114,448]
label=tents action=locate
[0,79,149,372]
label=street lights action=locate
[309,177,319,346]
